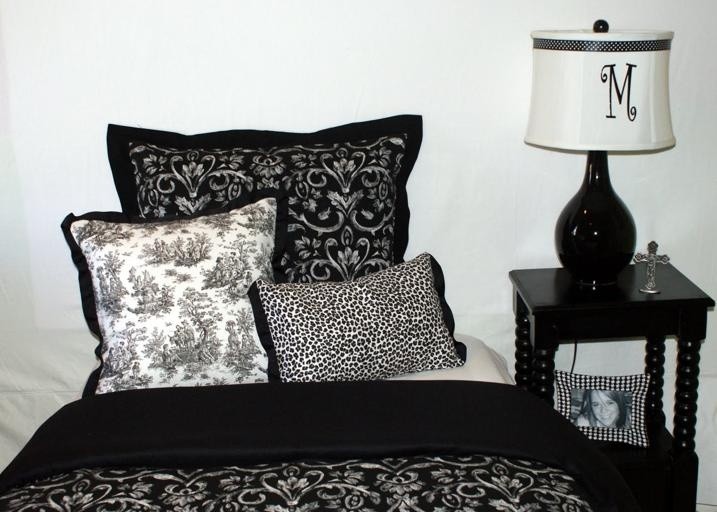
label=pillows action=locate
[61,187,289,393]
[247,252,467,382]
[105,115,425,285]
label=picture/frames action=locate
[552,369,649,449]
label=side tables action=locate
[508,262,717,512]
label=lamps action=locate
[524,20,676,285]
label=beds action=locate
[0,333,642,512]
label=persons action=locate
[568,387,628,428]
